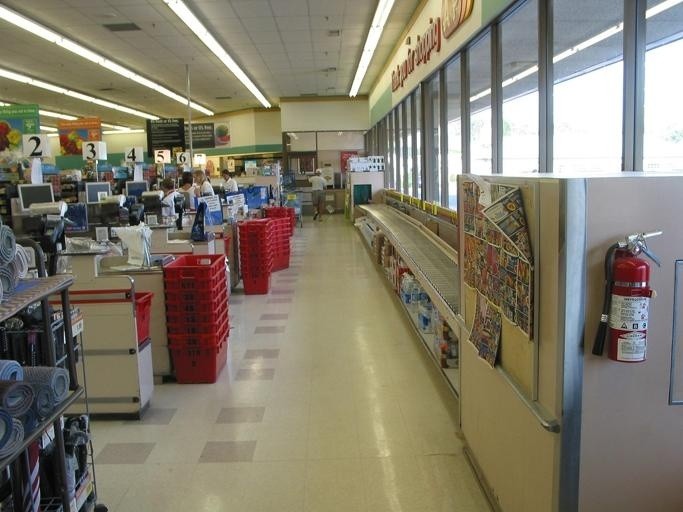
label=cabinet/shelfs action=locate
[0,162,185,228]
[0,237,108,512]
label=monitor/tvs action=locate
[178,178,194,188]
[126,180,149,196]
[158,179,175,189]
[17,183,54,211]
[86,182,111,204]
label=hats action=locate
[315,169,322,173]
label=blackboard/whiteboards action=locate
[184,123,215,148]
[147,118,185,158]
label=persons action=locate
[158,168,215,216]
[306,169,327,221]
[220,169,238,192]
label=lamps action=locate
[1,7,215,118]
[1,68,160,121]
[347,0,396,100]
[162,0,274,111]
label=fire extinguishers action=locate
[591,231,663,362]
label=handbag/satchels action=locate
[126,227,150,266]
[190,202,207,241]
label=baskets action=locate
[129,290,153,346]
[164,254,229,383]
[238,207,295,294]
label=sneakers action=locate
[313,212,323,222]
